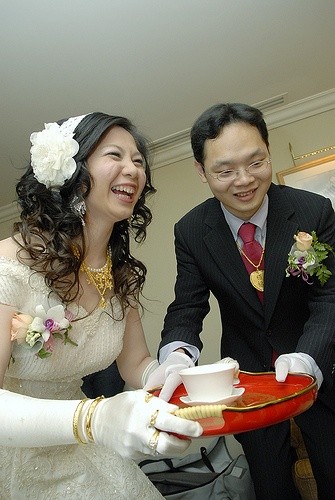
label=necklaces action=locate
[69,238,114,307]
[235,242,267,292]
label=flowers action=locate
[29,122,79,189]
[10,305,78,360]
[284,231,335,287]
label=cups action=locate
[179,363,236,401]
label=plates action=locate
[179,387,246,406]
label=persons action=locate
[144,104,335,500]
[0,113,203,500]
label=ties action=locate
[238,223,264,304]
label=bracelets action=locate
[85,396,102,448]
[72,399,89,448]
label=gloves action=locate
[139,350,196,403]
[0,357,241,459]
[275,351,324,401]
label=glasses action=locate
[201,155,273,183]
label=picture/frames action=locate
[275,153,335,212]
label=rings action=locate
[149,431,159,450]
[144,392,154,403]
[152,452,161,462]
[151,410,160,428]
